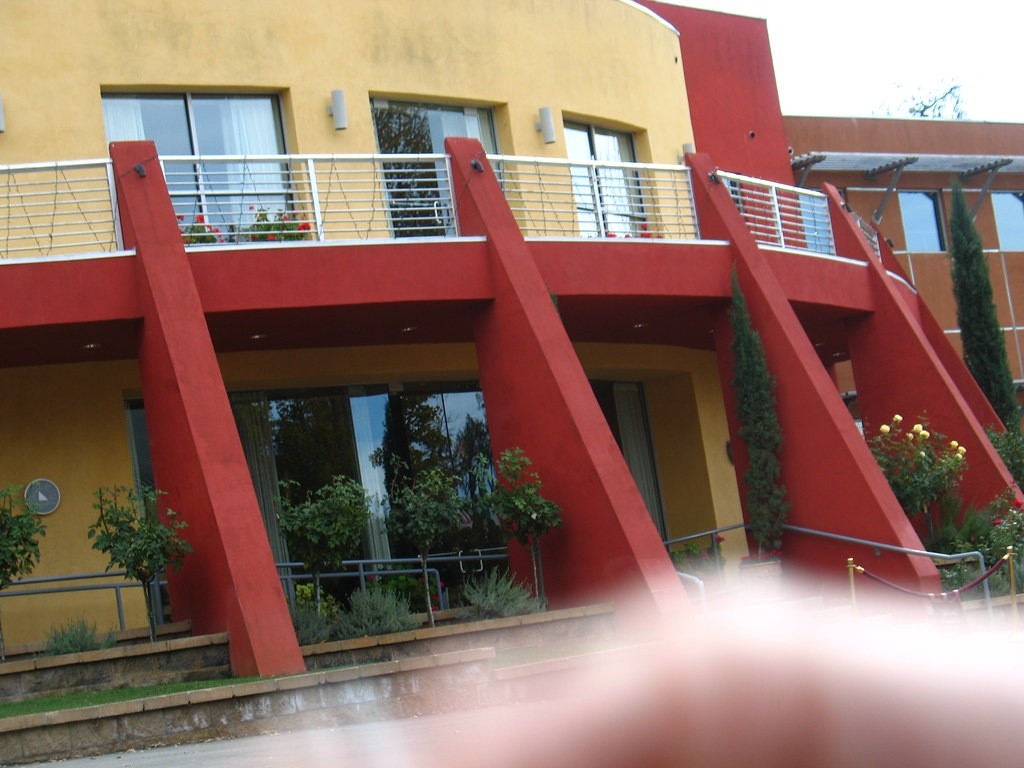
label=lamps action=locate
[328,90,348,131]
[535,106,557,145]
[677,143,693,165]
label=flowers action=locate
[245,205,314,242]
[174,214,224,246]
